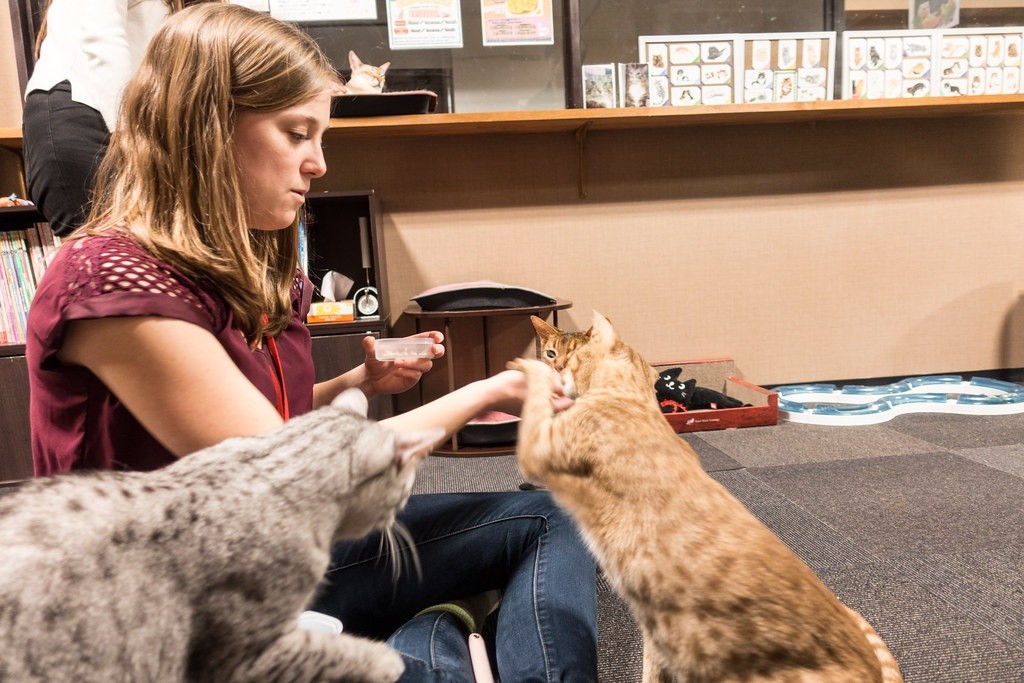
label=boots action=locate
[412,586,503,635]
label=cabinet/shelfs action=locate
[0,188,389,496]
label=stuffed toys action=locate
[655,366,754,414]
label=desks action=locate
[403,301,574,458]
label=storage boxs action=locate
[646,358,779,434]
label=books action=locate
[0,207,308,346]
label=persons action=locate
[26,5,600,682]
[20,0,221,240]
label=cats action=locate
[0,309,901,682]
[342,50,391,94]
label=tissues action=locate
[306,269,355,324]
